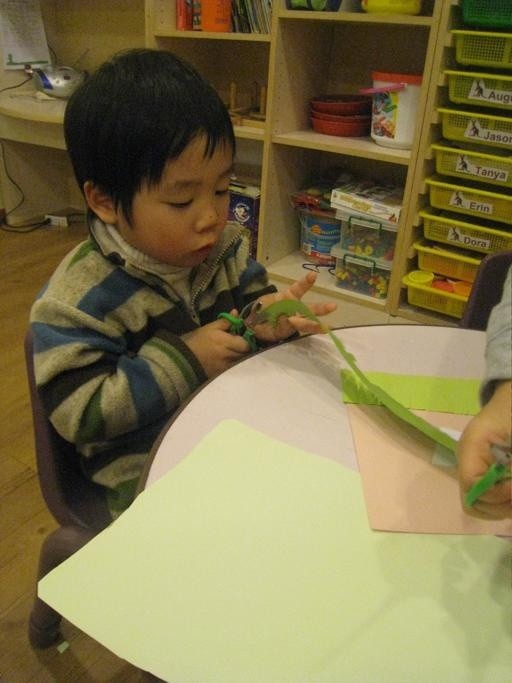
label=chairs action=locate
[24,326,137,649]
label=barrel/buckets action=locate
[358,68,423,148]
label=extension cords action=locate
[45,215,67,227]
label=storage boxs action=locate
[298,207,396,298]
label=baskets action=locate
[401,1,512,319]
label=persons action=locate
[458,263,510,521]
[28,46,336,520]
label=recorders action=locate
[33,64,91,98]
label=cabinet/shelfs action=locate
[144,0,443,312]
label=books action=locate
[175,0,273,33]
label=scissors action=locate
[216,300,271,356]
[464,435,512,508]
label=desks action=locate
[134,325,512,683]
[0,89,87,225]
